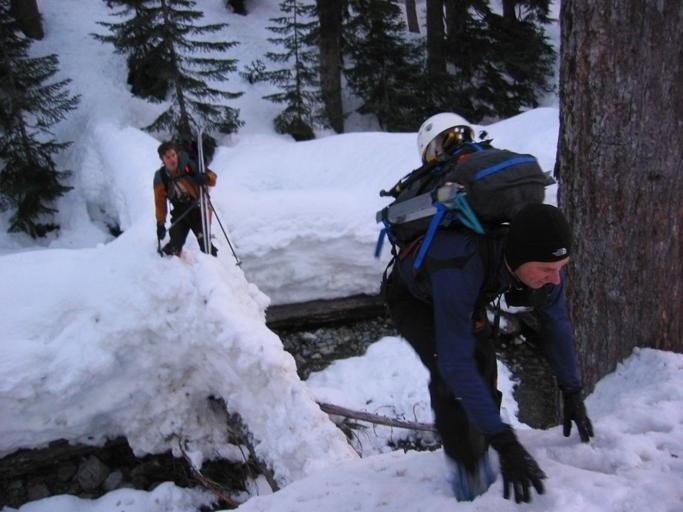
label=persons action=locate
[379,199,595,503]
[154,142,218,257]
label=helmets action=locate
[417,112,475,164]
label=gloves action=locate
[492,428,546,503]
[564,385,594,442]
[157,224,166,239]
[194,172,209,185]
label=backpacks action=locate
[375,148,546,302]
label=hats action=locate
[505,203,571,272]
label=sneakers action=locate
[458,451,491,500]
